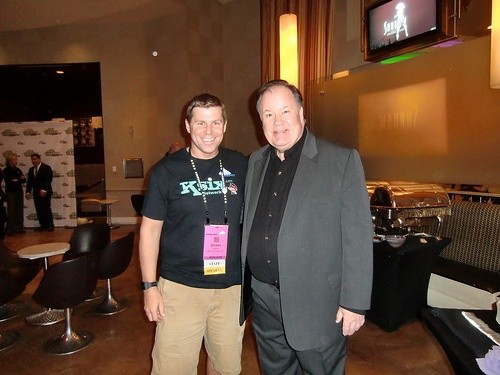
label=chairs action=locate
[132,194,144,217]
[0,199,135,355]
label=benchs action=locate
[423,201,500,310]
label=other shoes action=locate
[15,229,26,233]
[33,225,55,232]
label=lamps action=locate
[489,0,500,89]
[279,13,299,91]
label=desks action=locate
[368,229,451,333]
[16,242,73,326]
[98,198,121,230]
[420,304,500,375]
[445,188,500,204]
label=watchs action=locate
[141,281,157,290]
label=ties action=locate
[35,166,38,176]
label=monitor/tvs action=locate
[365,0,454,63]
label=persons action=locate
[3,155,26,235]
[139,94,249,375]
[239,80,373,375]
[26,153,53,232]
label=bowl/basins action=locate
[386,235,406,248]
